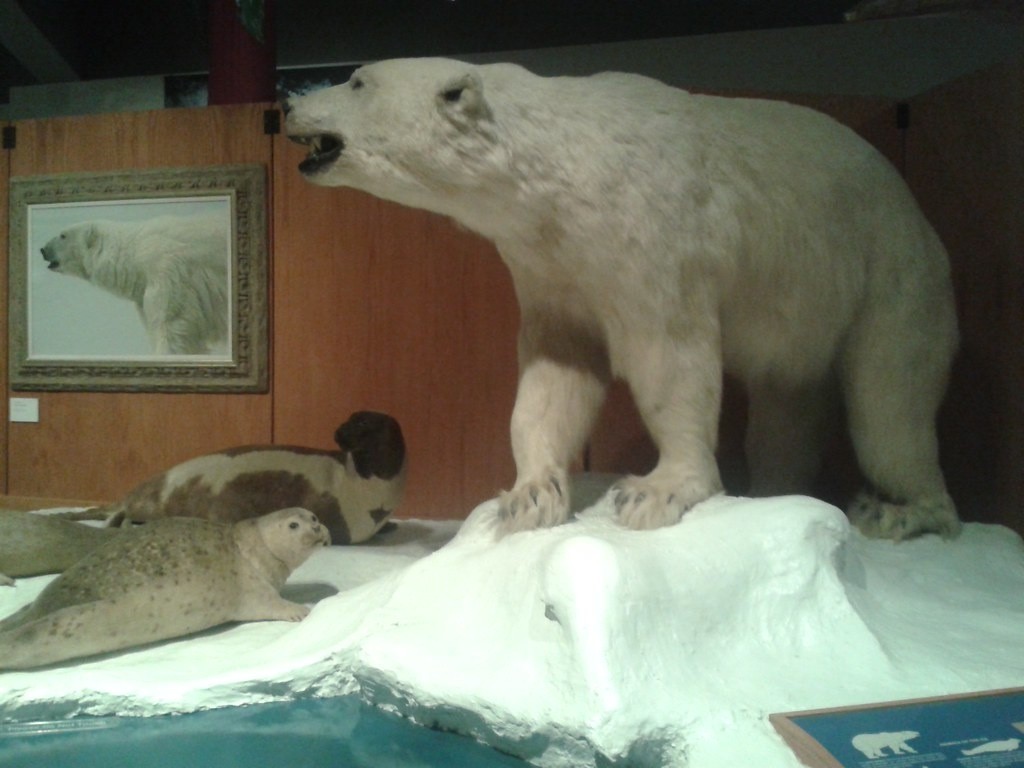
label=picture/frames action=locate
[8,162,269,394]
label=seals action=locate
[1,409,405,670]
